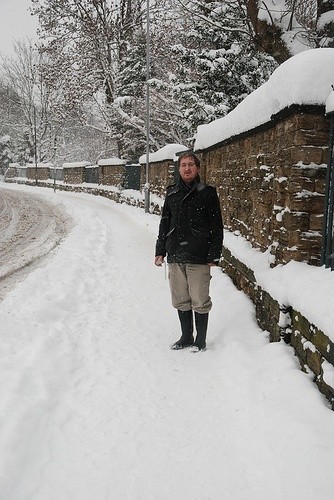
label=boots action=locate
[171,309,194,350]
[190,312,208,352]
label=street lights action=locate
[15,100,38,187]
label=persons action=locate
[154,152,224,352]
